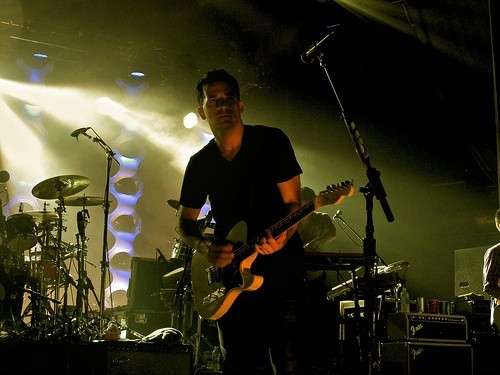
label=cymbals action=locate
[31,174,91,199]
[56,194,115,207]
[28,210,70,222]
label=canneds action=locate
[427,300,451,315]
[416,297,424,313]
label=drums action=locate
[31,250,56,284]
[6,213,36,252]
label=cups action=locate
[439,300,449,315]
[428,300,439,314]
[416,297,426,313]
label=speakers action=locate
[126,256,184,342]
[379,342,474,375]
[68,344,193,375]
[468,331,500,375]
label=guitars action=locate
[189,178,359,320]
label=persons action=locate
[482,209,500,336]
[0,200,30,331]
[178,68,308,375]
[297,187,336,277]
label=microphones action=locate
[333,209,342,220]
[299,31,335,65]
[71,127,91,137]
[157,248,172,270]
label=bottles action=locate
[400,288,410,313]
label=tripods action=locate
[0,135,144,343]
